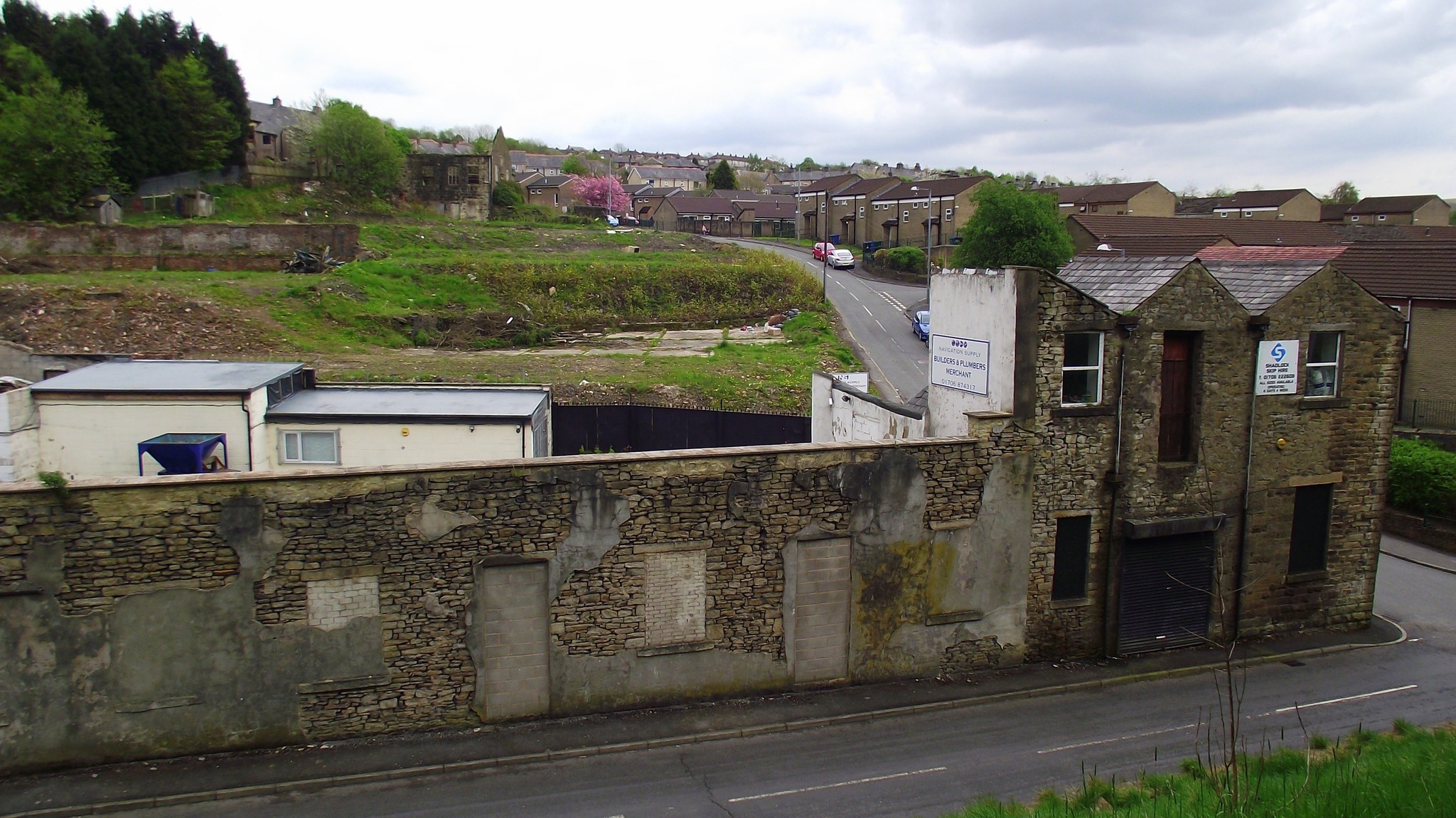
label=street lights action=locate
[910,185,931,305]
[600,156,612,215]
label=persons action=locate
[702,225,707,235]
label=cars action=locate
[623,216,639,226]
[911,309,929,340]
[813,242,836,261]
[605,215,619,227]
[827,249,855,269]
